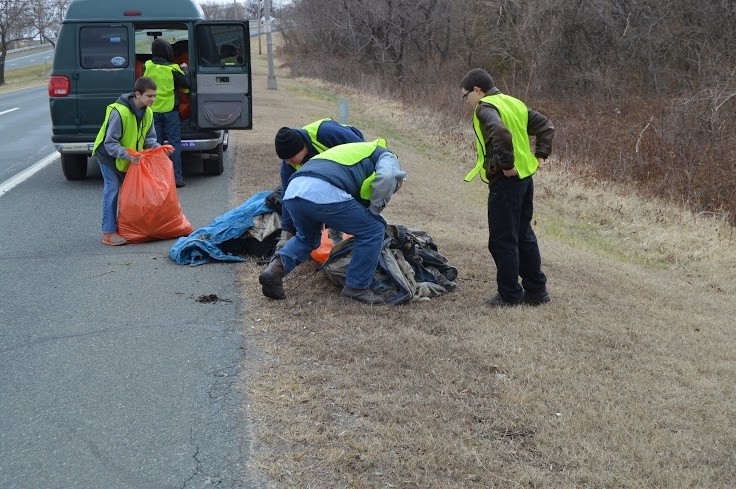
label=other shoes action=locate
[484,294,523,306]
[523,294,552,306]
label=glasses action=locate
[462,89,473,99]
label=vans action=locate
[46,0,252,180]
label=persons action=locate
[275,117,366,253]
[259,139,406,304]
[143,39,190,188]
[93,78,173,245]
[461,69,555,308]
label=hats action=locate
[275,127,304,160]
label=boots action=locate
[341,284,385,306]
[259,257,285,299]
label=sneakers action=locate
[102,233,127,245]
